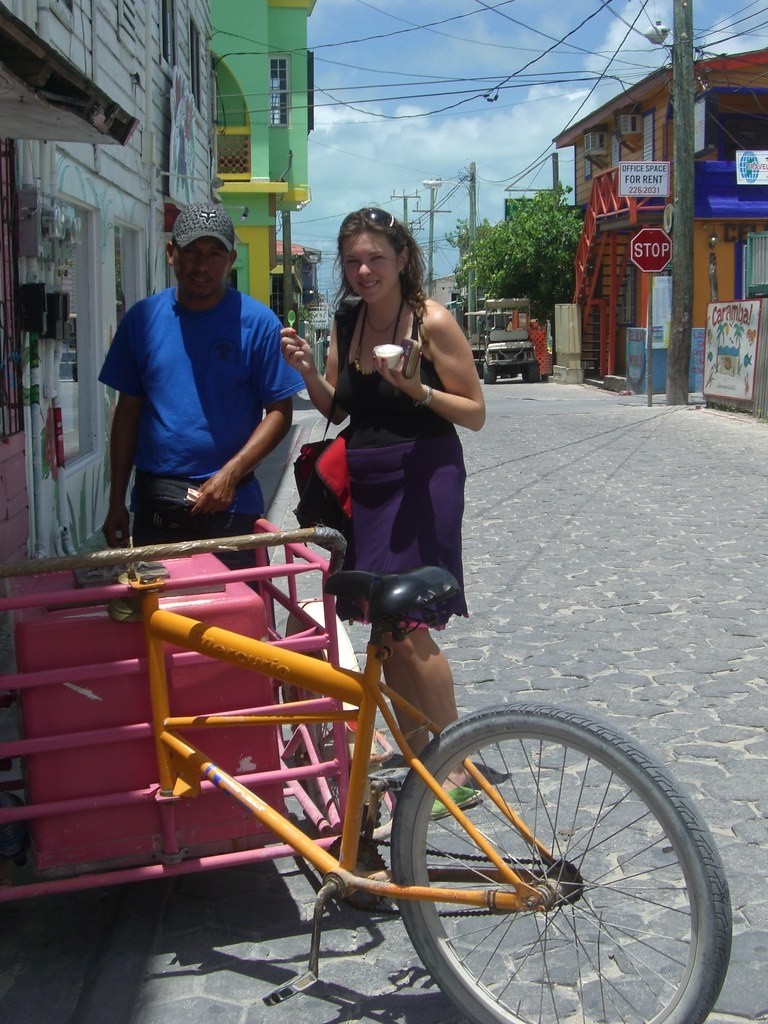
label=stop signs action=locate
[631,228,675,272]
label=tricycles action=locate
[0,521,739,1024]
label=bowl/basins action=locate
[373,344,405,369]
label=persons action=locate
[279,207,485,820]
[97,204,308,631]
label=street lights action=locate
[643,1,709,408]
[421,162,484,370]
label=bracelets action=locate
[412,385,433,407]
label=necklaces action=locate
[354,296,405,376]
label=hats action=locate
[172,199,235,255]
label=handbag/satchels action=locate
[291,426,355,568]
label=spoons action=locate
[287,310,295,338]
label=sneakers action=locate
[428,784,484,821]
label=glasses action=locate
[343,208,406,239]
